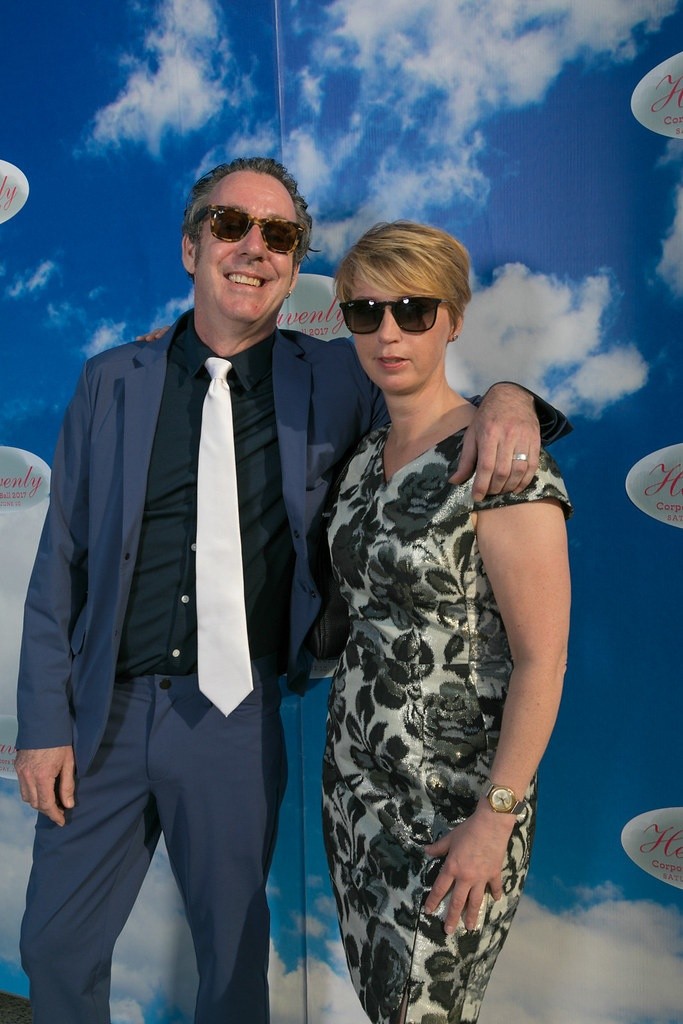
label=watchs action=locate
[479,779,527,815]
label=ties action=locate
[195,357,255,716]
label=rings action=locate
[512,454,528,463]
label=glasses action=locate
[194,204,302,255]
[339,296,451,334]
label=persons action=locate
[322,222,572,1024]
[13,156,575,1024]
